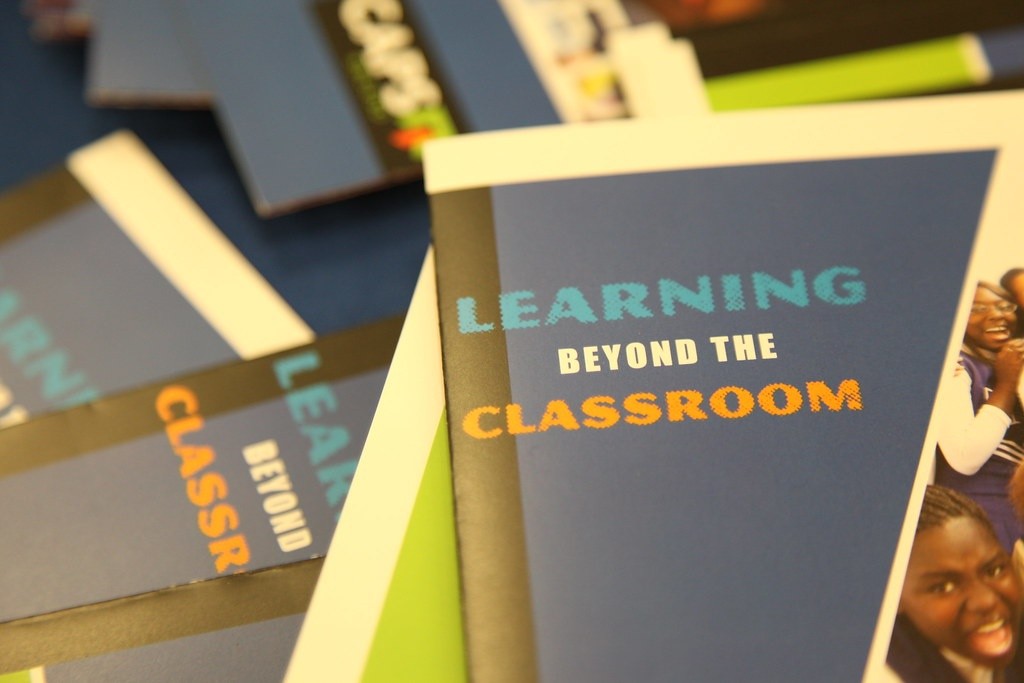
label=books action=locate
[0,1,1024,683]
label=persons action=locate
[1000,268,1024,407]
[880,486,1023,683]
[932,281,1024,553]
